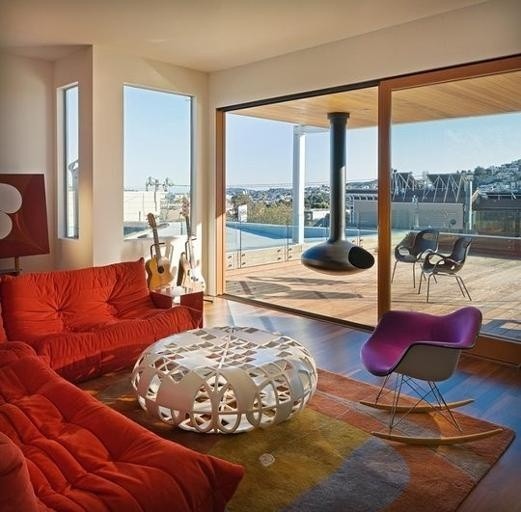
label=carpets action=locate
[78,367,514,512]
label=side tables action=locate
[150,286,203,328]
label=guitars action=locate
[145,213,173,290]
[179,198,206,295]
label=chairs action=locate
[418,237,472,303]
[359,307,503,446]
[391,229,439,289]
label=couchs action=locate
[0,257,194,384]
[0,341,245,512]
